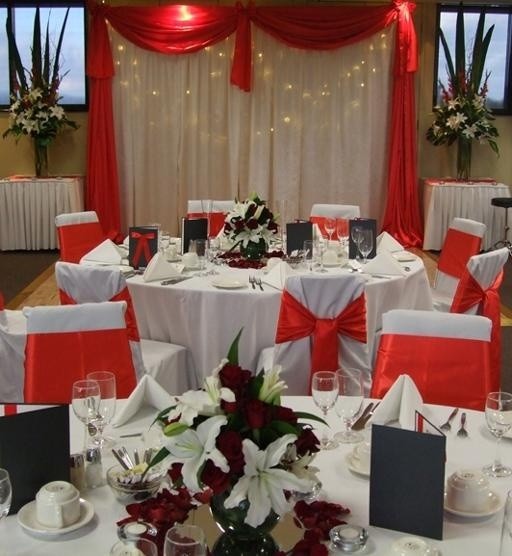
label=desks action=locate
[1,175,87,256]
[422,177,509,251]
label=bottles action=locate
[70,447,103,490]
[326,524,372,556]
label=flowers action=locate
[98,327,354,554]
[1,5,79,172]
[427,2,502,177]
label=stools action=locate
[485,197,512,259]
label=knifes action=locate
[349,401,374,428]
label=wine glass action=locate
[190,236,223,279]
[198,196,214,236]
[301,238,319,274]
[321,213,375,274]
[276,198,289,253]
[0,467,12,519]
[105,462,163,538]
[482,390,512,477]
[309,365,366,449]
[71,369,116,456]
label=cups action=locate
[446,469,492,512]
[109,536,158,556]
[500,489,512,555]
[33,479,82,528]
[162,524,208,556]
[352,440,371,469]
[387,534,444,556]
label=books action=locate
[285,218,313,261]
[348,216,377,260]
[180,217,208,257]
[128,225,159,271]
[367,408,448,543]
[0,401,71,517]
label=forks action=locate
[248,273,267,293]
[438,409,471,438]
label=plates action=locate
[345,452,371,476]
[211,273,247,289]
[16,498,96,535]
[444,485,505,519]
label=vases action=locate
[457,136,472,182]
[32,138,48,177]
